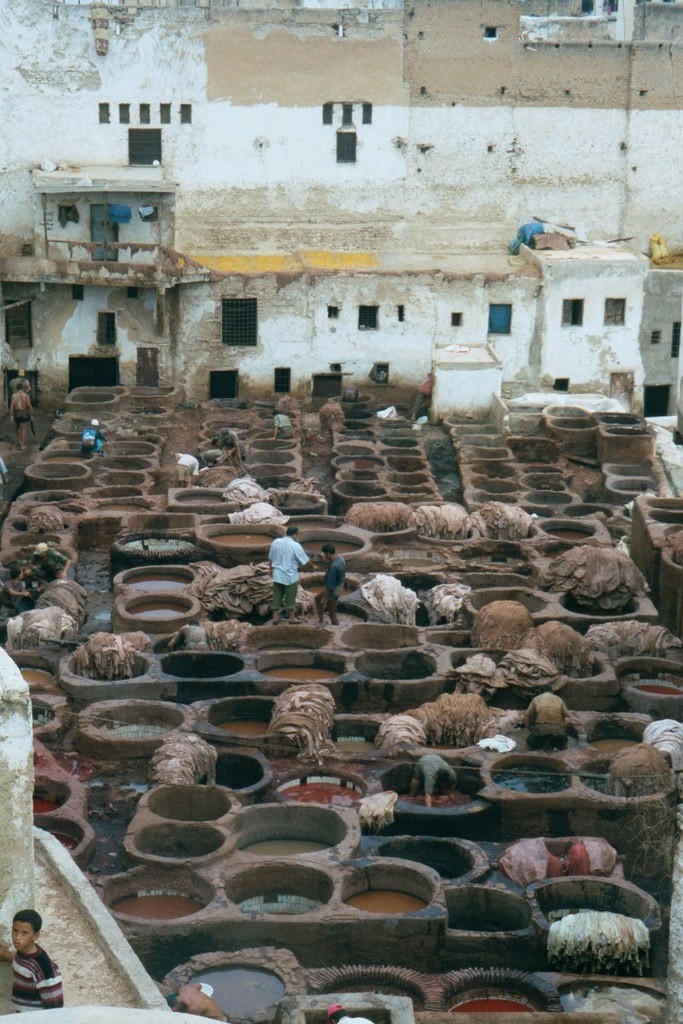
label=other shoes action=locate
[289,619,302,624]
[274,617,280,625]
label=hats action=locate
[18,369,24,376]
[222,429,230,439]
[91,419,99,425]
[33,543,47,554]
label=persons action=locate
[409,372,432,419]
[0,909,63,1015]
[525,684,571,751]
[162,981,227,1022]
[10,383,34,450]
[168,619,214,654]
[325,1003,378,1024]
[271,410,297,440]
[410,754,458,808]
[9,368,32,397]
[268,525,322,626]
[0,457,8,494]
[23,543,72,582]
[313,544,346,627]
[172,426,249,488]
[1,566,41,612]
[80,418,108,452]
